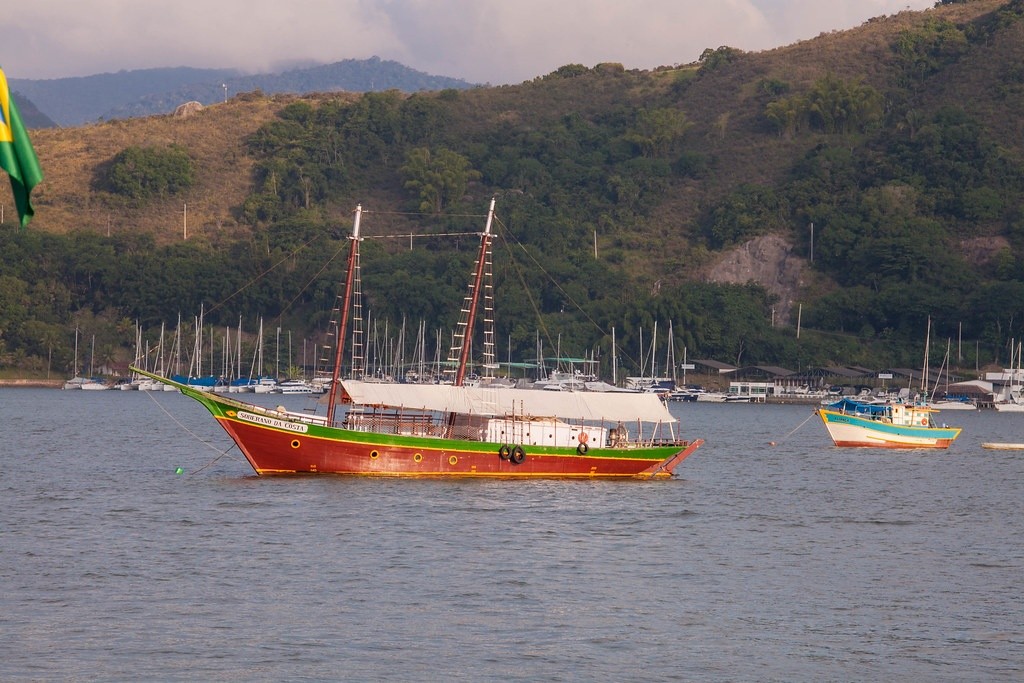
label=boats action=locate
[127,196,702,481]
[814,388,963,449]
[697,387,728,403]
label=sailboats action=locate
[81,333,112,389]
[62,323,87,390]
[909,314,1024,412]
[114,303,698,403]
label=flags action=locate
[0,66,44,233]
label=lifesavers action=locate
[512,447,525,464]
[578,443,589,454]
[499,445,512,459]
